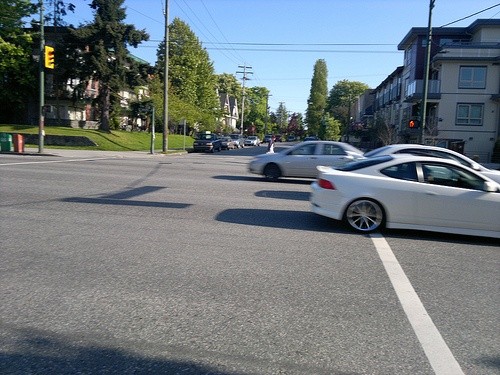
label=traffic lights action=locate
[408,120,419,128]
[45,45,56,69]
[43,105,53,116]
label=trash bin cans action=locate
[14,134,25,153]
[0,132,12,152]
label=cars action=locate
[193,130,262,153]
[308,143,500,239]
[263,133,283,142]
[247,141,366,183]
[304,136,317,141]
[286,135,296,141]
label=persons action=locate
[425,169,435,182]
[268,135,275,152]
